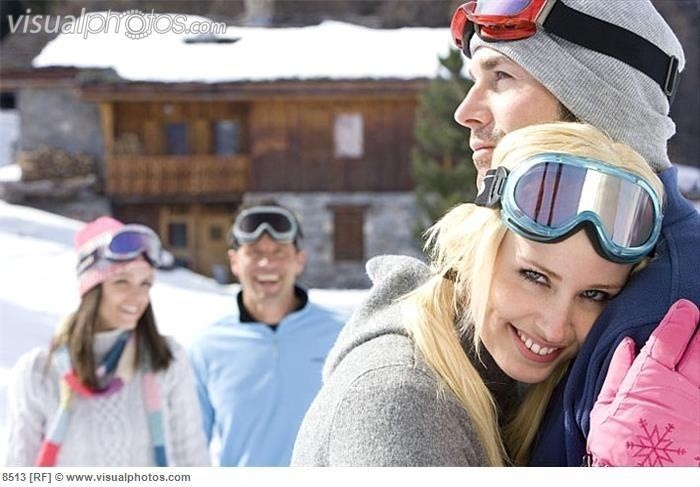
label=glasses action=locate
[471,153,665,264]
[449,0,679,100]
[78,224,174,270]
[226,206,305,247]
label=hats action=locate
[469,0,686,171]
[77,217,154,296]
[225,199,303,253]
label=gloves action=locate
[581,299,700,467]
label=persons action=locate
[0,216,212,465]
[451,1,700,466]
[185,198,351,465]
[288,122,700,467]
